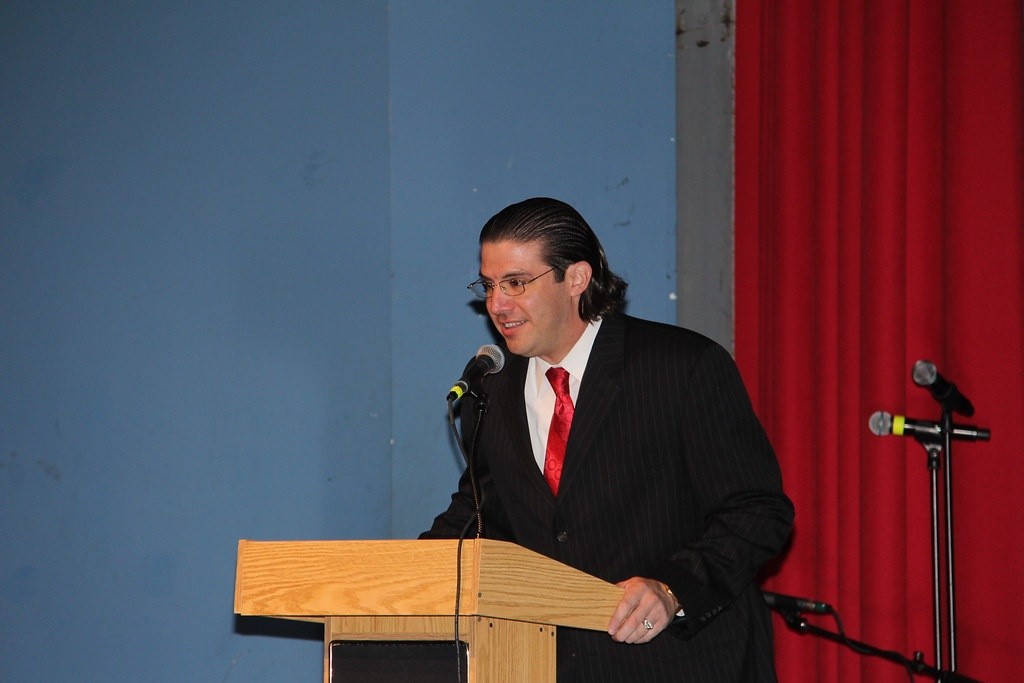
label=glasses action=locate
[466,266,558,299]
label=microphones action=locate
[760,590,831,614]
[912,360,975,418]
[446,344,506,402]
[869,411,991,442]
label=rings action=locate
[642,618,654,629]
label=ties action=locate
[543,366,576,498]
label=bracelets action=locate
[667,588,678,603]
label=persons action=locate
[420,198,796,683]
